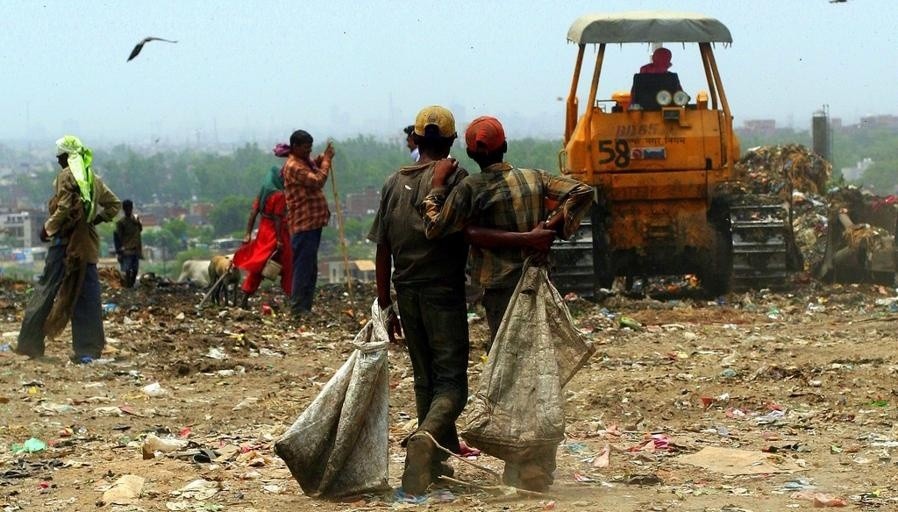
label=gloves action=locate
[40,227,51,242]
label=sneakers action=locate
[401,431,454,498]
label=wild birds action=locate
[126,37,179,62]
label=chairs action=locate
[631,74,678,112]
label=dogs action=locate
[206,254,243,306]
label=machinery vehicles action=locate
[540,11,794,304]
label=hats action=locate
[466,116,505,152]
[415,105,456,137]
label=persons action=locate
[9,136,121,364]
[114,199,142,289]
[403,125,450,161]
[640,47,672,74]
[418,115,595,493]
[283,129,335,312]
[232,165,293,310]
[367,105,557,496]
